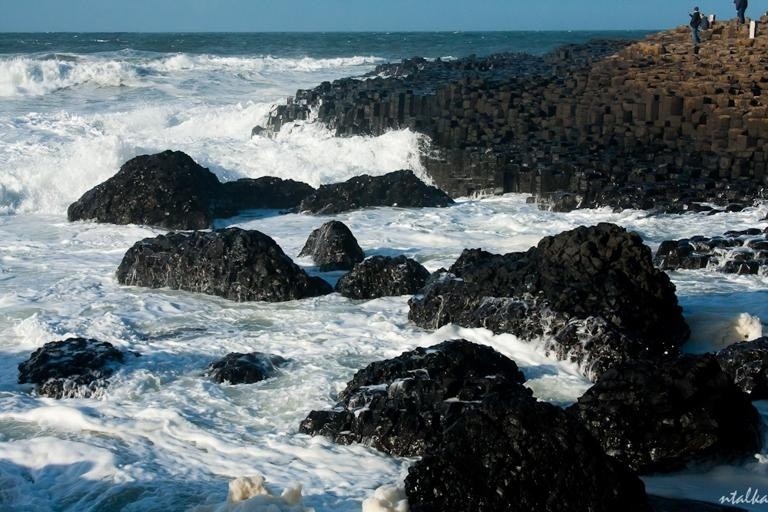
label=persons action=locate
[734,0,749,24]
[687,6,702,45]
[699,14,710,31]
